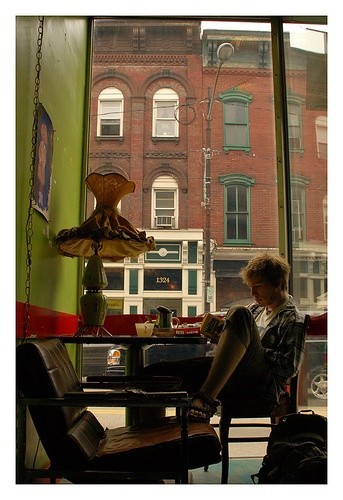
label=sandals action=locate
[188,393,217,423]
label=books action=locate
[201,314,224,339]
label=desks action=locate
[59,333,209,472]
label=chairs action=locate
[220,313,312,485]
[17,338,223,484]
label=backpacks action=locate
[251,410,327,484]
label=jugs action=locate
[156,305,179,337]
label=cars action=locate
[102,298,329,397]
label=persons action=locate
[188,254,307,419]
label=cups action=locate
[135,323,154,337]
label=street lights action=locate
[203,42,236,313]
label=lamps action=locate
[57,171,156,338]
